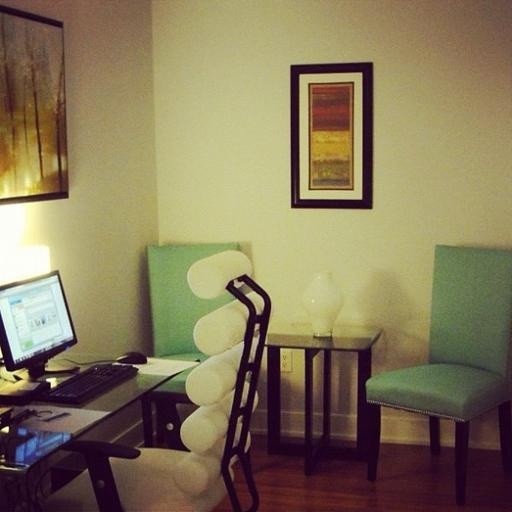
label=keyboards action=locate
[43,363,139,409]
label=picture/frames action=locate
[290,62,372,209]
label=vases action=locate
[302,272,343,339]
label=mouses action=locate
[117,351,147,365]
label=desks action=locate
[262,335,376,477]
[0,350,198,511]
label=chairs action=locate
[46,251,270,512]
[365,244,512,503]
[141,244,242,443]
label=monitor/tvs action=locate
[0,270,78,385]
